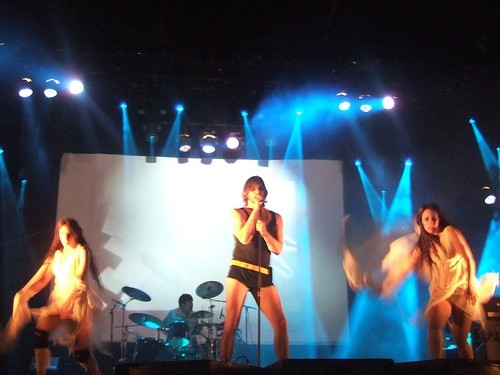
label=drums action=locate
[132,337,168,362]
[166,320,190,348]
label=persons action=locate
[1,216,103,375]
[220,175,289,367]
[381,202,479,359]
[162,293,204,359]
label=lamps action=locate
[16,69,85,99]
[177,127,242,154]
[334,88,396,112]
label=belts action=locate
[231,259,269,275]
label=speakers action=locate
[110,353,500,375]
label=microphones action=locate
[259,200,265,206]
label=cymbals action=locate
[122,286,151,302]
[191,311,214,318]
[128,313,163,329]
[196,281,224,300]
